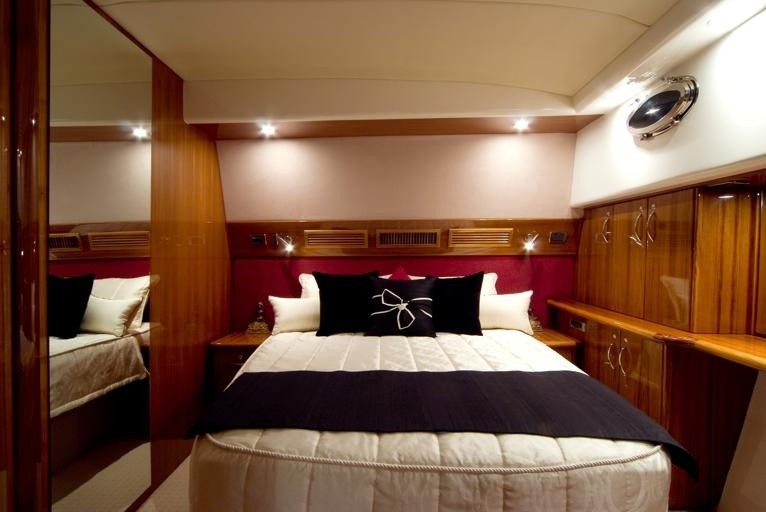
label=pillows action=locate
[47,273,151,339]
[267,270,535,340]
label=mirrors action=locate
[49,0,153,511]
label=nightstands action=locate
[209,332,270,398]
[534,327,582,366]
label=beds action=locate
[190,332,676,512]
[46,323,152,468]
